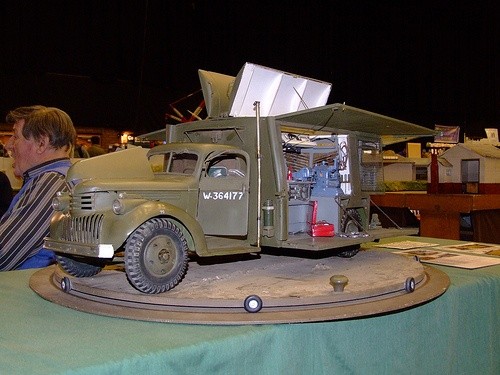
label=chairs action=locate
[205,166,227,177]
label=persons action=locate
[0,104,76,273]
[77,143,91,157]
[89,135,106,158]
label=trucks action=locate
[43,62,440,294]
